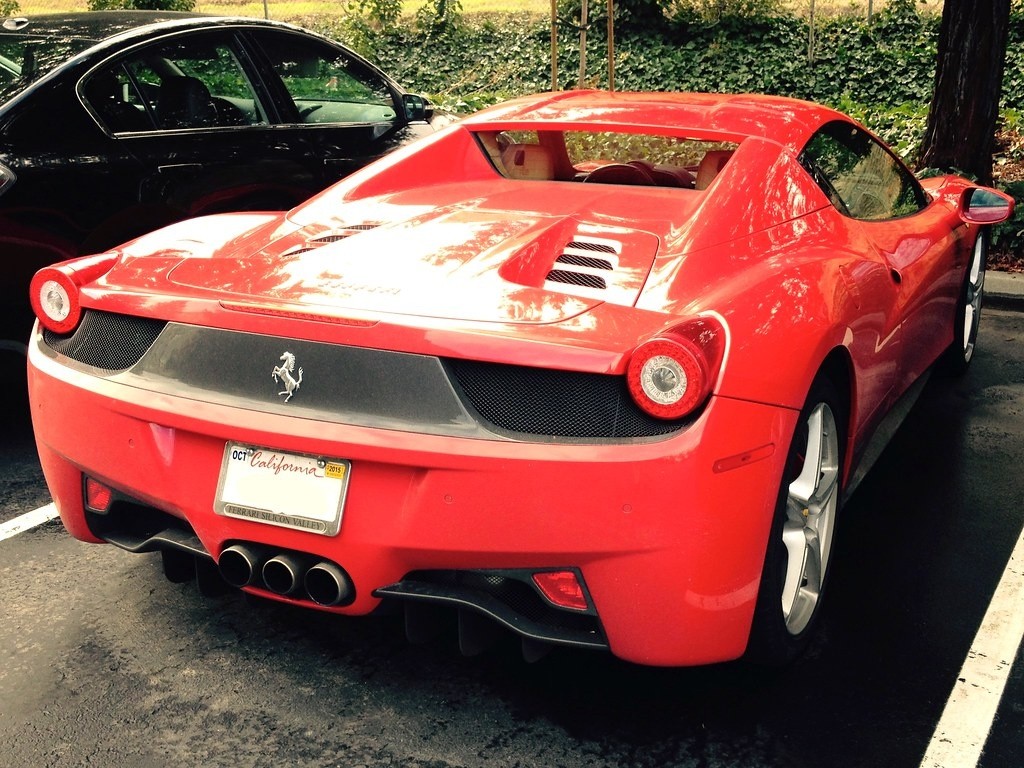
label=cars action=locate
[25,88,1013,676]
[1,11,452,438]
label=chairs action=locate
[155,76,219,127]
[81,75,154,133]
[500,144,554,181]
[695,150,734,189]
[582,164,656,187]
